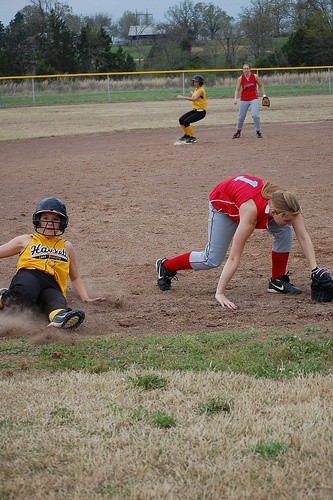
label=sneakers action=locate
[267,271,302,294]
[46,307,85,331]
[179,134,190,141]
[185,137,197,143]
[156,257,178,292]
[0,288,8,309]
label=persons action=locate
[176,74,206,145]
[231,63,271,139]
[156,174,330,309]
[0,197,107,332]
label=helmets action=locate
[191,75,203,85]
[32,197,68,236]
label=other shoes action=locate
[256,133,262,137]
[233,132,240,138]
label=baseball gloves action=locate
[307,266,333,300]
[262,95,270,107]
